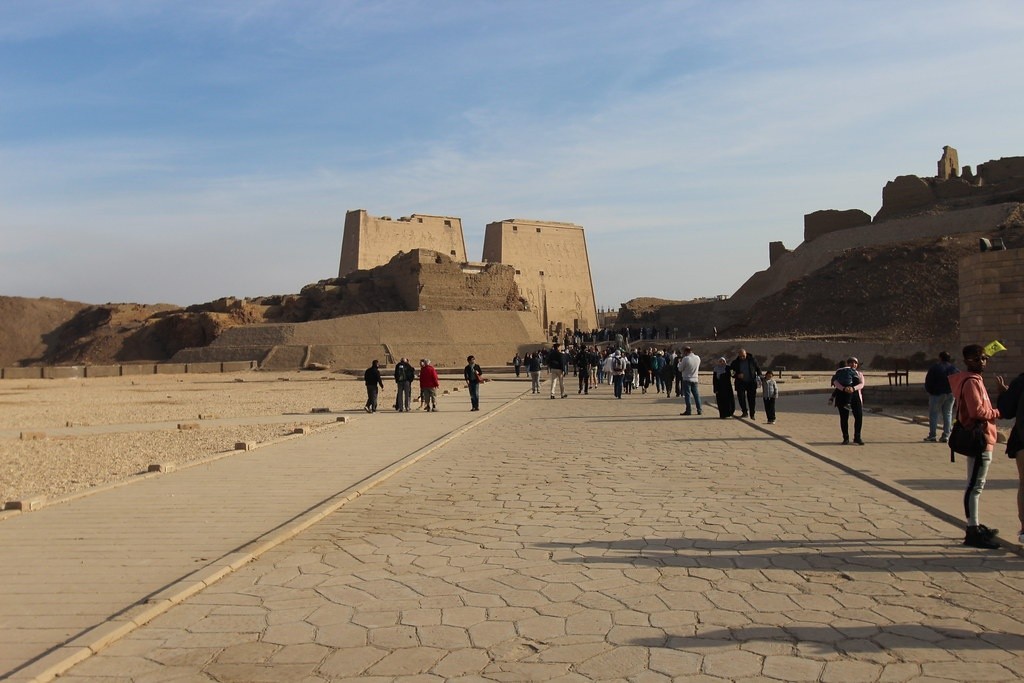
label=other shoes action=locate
[1018,532,1024,543]
[680,411,691,415]
[939,437,949,443]
[962,530,1000,549]
[750,415,755,420]
[364,375,683,414]
[844,404,852,411]
[924,436,936,443]
[740,413,748,418]
[698,412,702,415]
[828,398,834,405]
[965,523,999,538]
[768,420,775,424]
[853,437,865,445]
[842,437,849,444]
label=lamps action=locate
[980,238,1006,251]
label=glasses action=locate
[967,356,989,362]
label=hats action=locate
[838,360,847,367]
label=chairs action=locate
[888,359,908,386]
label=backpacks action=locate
[613,356,622,372]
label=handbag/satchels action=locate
[947,420,987,458]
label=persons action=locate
[394,358,414,412]
[524,343,685,399]
[996,373,1024,541]
[761,371,778,424]
[828,357,864,445]
[418,359,439,412]
[713,327,717,340]
[665,328,669,340]
[924,352,960,442]
[947,344,1000,549]
[464,356,482,411]
[364,360,384,412]
[713,358,735,419]
[550,326,658,347]
[512,353,522,377]
[730,349,763,420]
[677,346,702,415]
